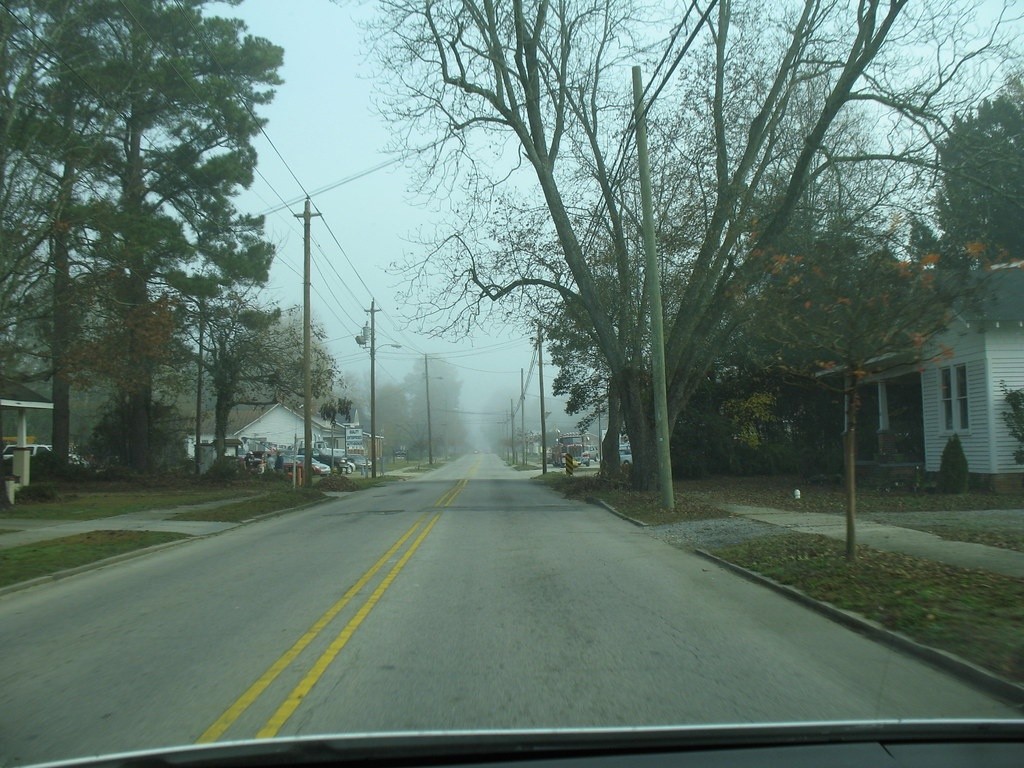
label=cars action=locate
[588,444,599,461]
[620,446,633,466]
[2,442,91,469]
[243,448,371,479]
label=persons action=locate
[243,450,285,476]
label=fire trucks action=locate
[551,429,591,467]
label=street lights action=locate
[370,342,404,480]
[424,375,444,465]
